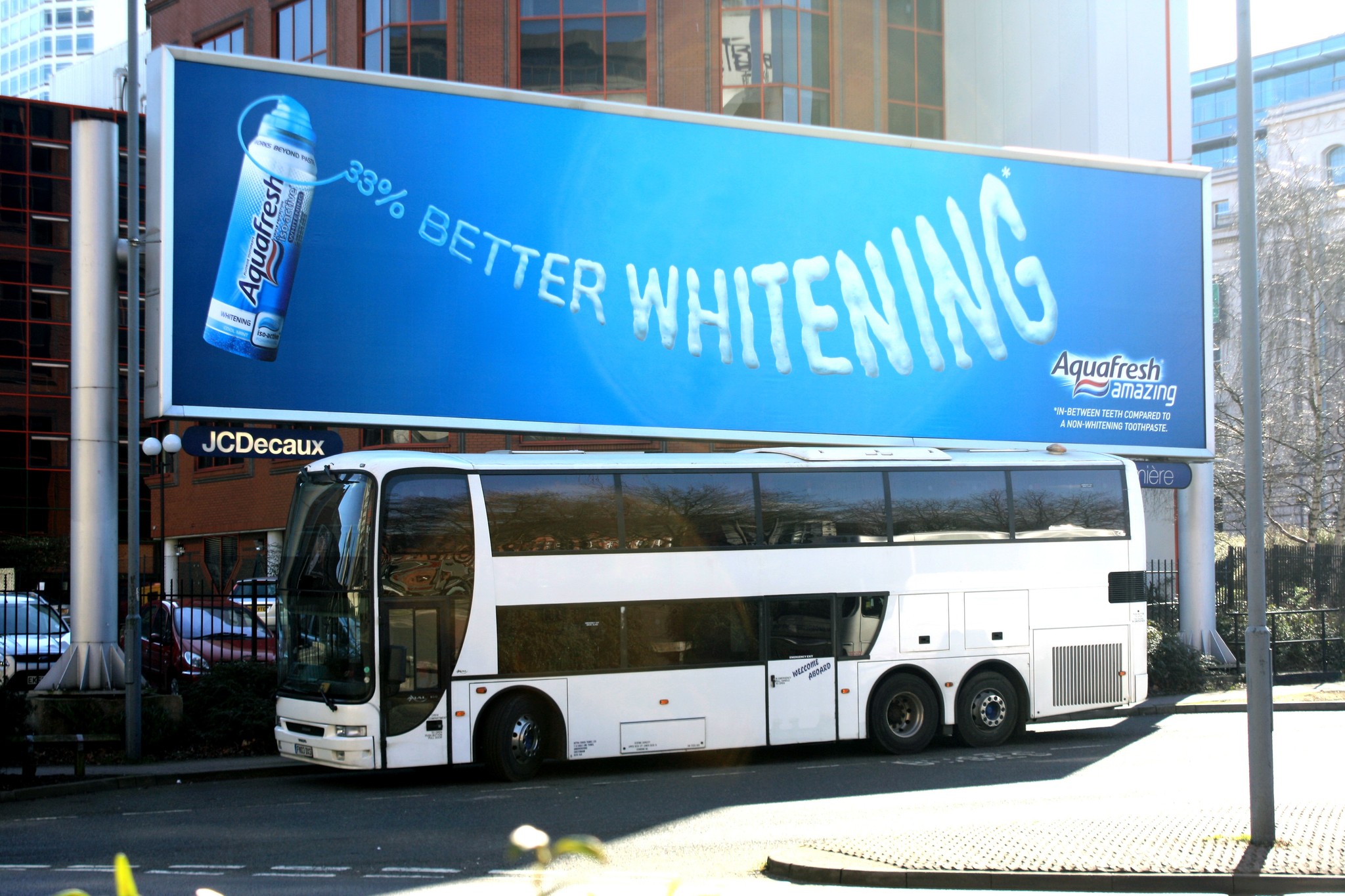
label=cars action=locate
[119,595,288,696]
[296,613,360,659]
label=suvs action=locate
[227,576,291,634]
[0,589,70,689]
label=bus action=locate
[768,525,1125,658]
[273,442,1149,782]
